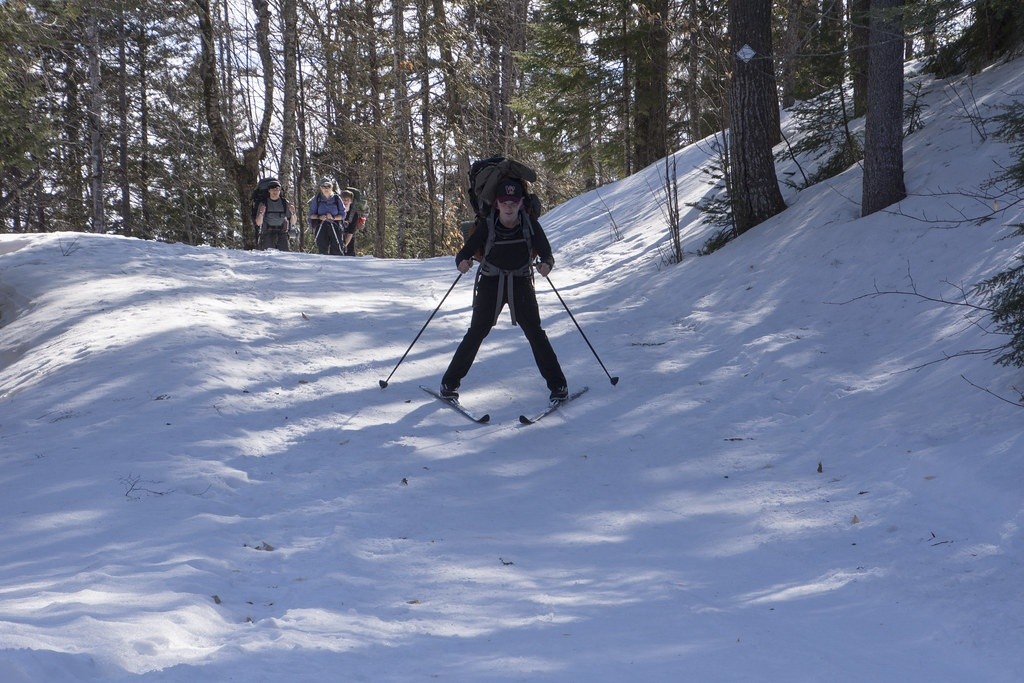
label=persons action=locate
[256,181,297,252]
[340,189,359,257]
[439,177,569,402]
[308,177,347,256]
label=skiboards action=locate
[418,383,591,425]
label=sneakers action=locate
[550,385,568,401]
[439,380,459,399]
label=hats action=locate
[340,190,354,200]
[495,180,523,203]
[267,180,281,189]
[319,177,333,187]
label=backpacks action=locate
[249,177,284,202]
[461,156,542,261]
[346,187,370,234]
